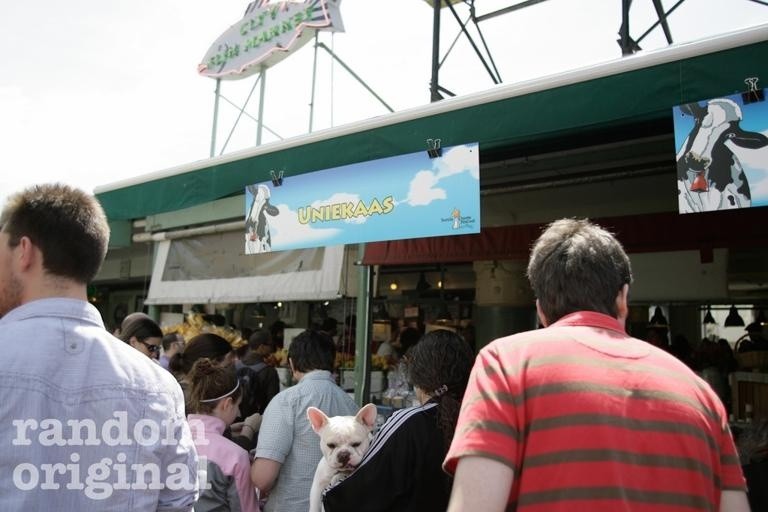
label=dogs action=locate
[306,402,377,512]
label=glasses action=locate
[142,342,161,352]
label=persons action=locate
[108,303,498,511]
[0,182,203,512]
[441,215,752,510]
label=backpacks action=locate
[235,360,266,417]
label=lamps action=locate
[379,268,447,291]
[629,301,768,326]
[436,303,452,322]
[251,303,265,318]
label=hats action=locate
[250,330,273,345]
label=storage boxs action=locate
[275,367,292,392]
[339,367,387,392]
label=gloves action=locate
[240,413,262,440]
